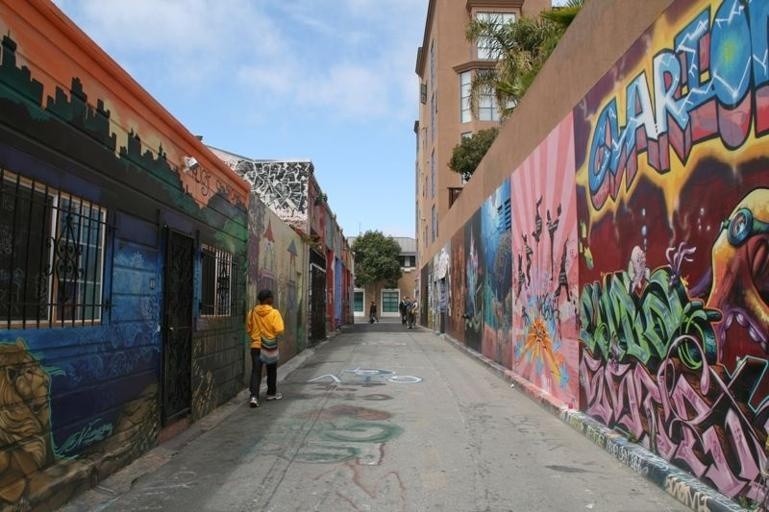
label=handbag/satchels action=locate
[260,336,280,364]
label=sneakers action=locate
[250,397,260,407]
[266,393,282,399]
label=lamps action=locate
[183,156,199,172]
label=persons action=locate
[398,295,419,329]
[368,300,379,322]
[245,288,285,407]
